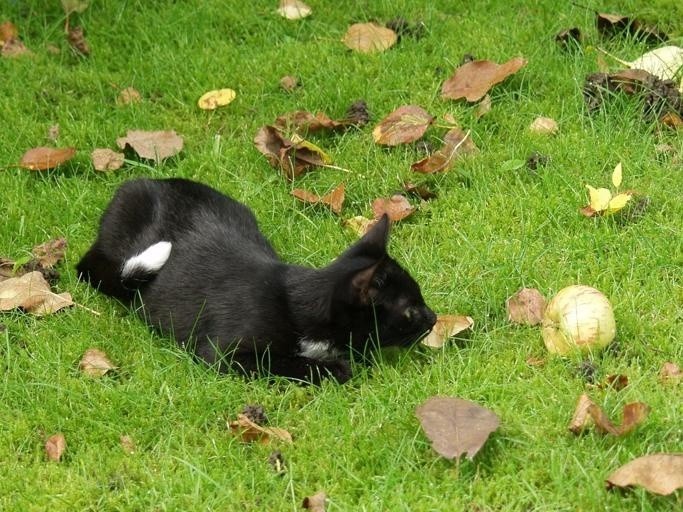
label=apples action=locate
[538,285,616,359]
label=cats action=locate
[72,176,439,387]
